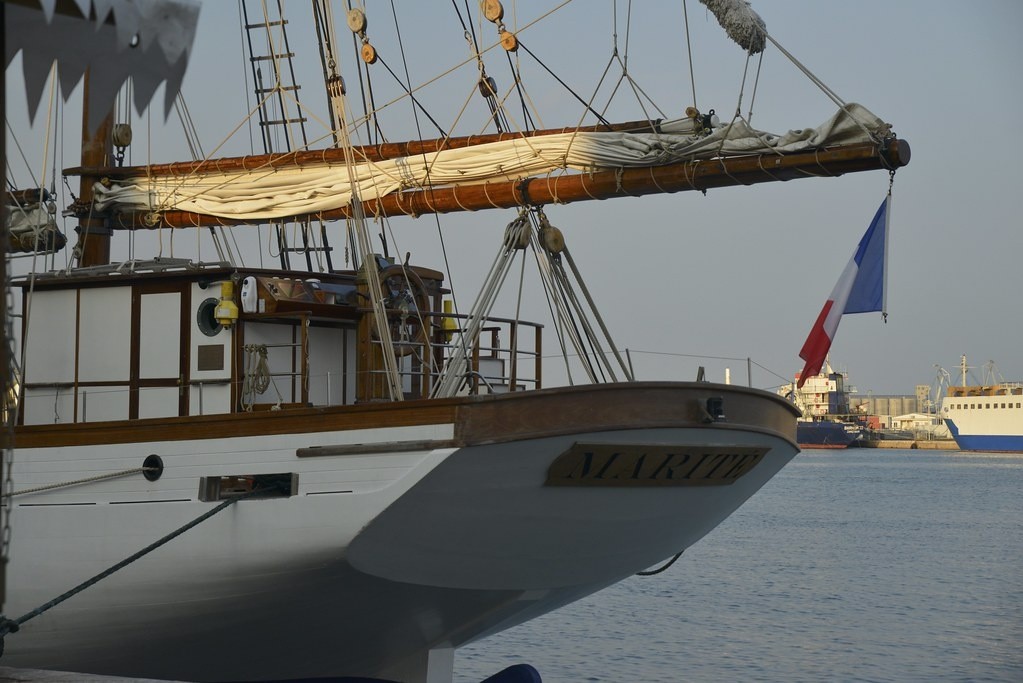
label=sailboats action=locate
[0,1,910,683]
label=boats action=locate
[940,353,1022,453]
[776,352,868,449]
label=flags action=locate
[796,196,890,388]
